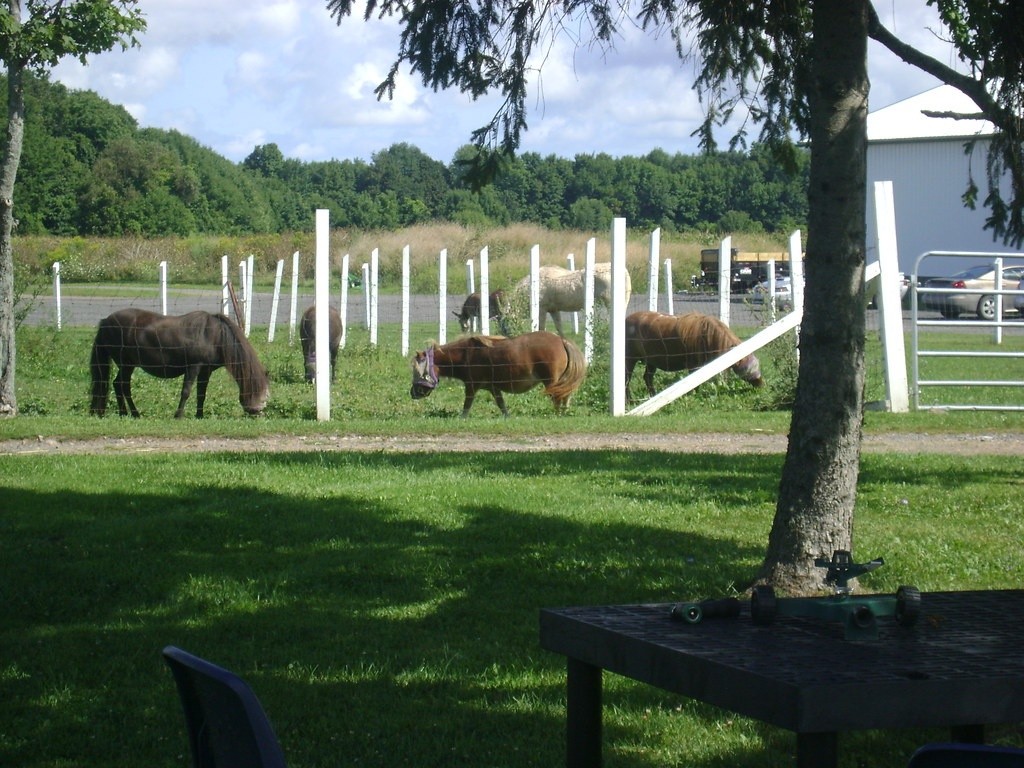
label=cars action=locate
[922,264,1024,321]
[752,271,908,312]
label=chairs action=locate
[161,644,287,768]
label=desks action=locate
[537,589,1024,768]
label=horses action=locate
[450,259,632,336]
[298,294,344,387]
[86,306,272,421]
[624,310,767,406]
[409,329,587,423]
